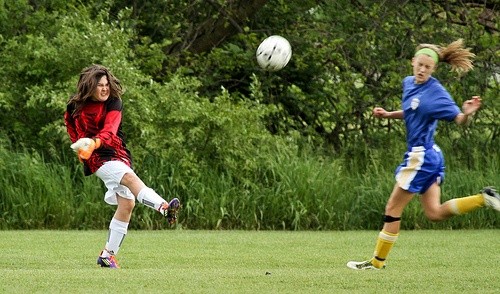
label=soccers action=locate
[256,34,293,72]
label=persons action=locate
[346,38,500,272]
[62,66,179,270]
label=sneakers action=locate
[162,198,180,224]
[347,260,386,271]
[482,186,500,211]
[96,251,121,269]
